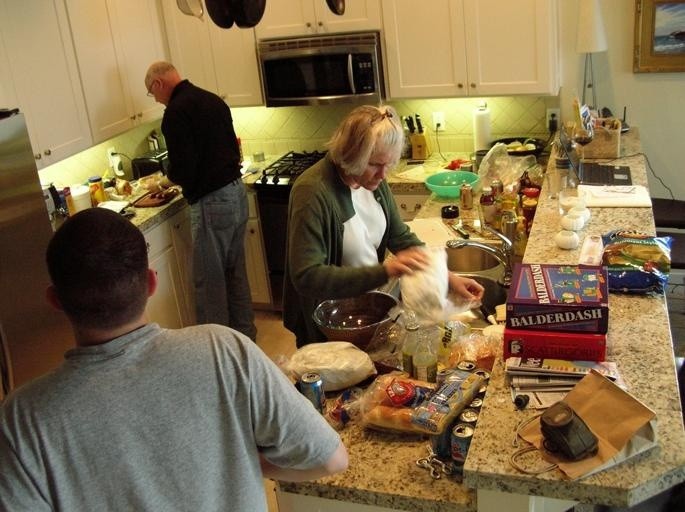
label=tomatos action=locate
[448,160,459,169]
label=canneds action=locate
[430,361,491,476]
[300,371,327,417]
[491,180,503,197]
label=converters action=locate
[548,119,557,131]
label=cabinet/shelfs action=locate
[159,1,262,112]
[394,194,429,222]
[242,193,271,309]
[65,0,168,144]
[254,0,379,37]
[1,0,92,171]
[374,0,559,100]
[142,211,192,333]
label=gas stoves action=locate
[252,150,330,186]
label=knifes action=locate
[404,114,424,133]
[448,222,470,237]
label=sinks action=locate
[446,274,507,330]
[443,242,505,272]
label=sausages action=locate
[148,185,175,199]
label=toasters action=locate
[131,148,168,177]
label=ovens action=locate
[254,186,292,308]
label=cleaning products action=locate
[513,216,528,257]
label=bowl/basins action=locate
[312,291,401,350]
[425,171,480,198]
[490,137,549,160]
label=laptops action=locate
[559,122,632,186]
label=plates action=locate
[482,324,506,336]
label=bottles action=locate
[88,177,106,205]
[413,329,437,384]
[480,171,540,256]
[402,312,422,377]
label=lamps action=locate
[574,1,607,110]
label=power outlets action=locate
[546,108,561,130]
[432,113,445,131]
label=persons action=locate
[282,103,486,351]
[144,60,258,345]
[0,206,351,512]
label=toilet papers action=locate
[69,184,92,213]
[472,107,492,159]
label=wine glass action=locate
[573,121,594,161]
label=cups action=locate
[559,187,588,215]
[554,139,570,168]
[253,152,265,162]
[477,149,491,171]
[546,171,567,199]
[461,163,473,173]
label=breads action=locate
[362,372,482,436]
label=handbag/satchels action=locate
[509,369,657,482]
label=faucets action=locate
[445,224,514,287]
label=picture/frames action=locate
[632,1,685,76]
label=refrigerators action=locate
[1,106,80,403]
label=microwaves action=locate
[256,31,383,108]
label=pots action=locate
[204,0,267,30]
[326,1,346,16]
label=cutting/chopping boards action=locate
[134,189,179,208]
